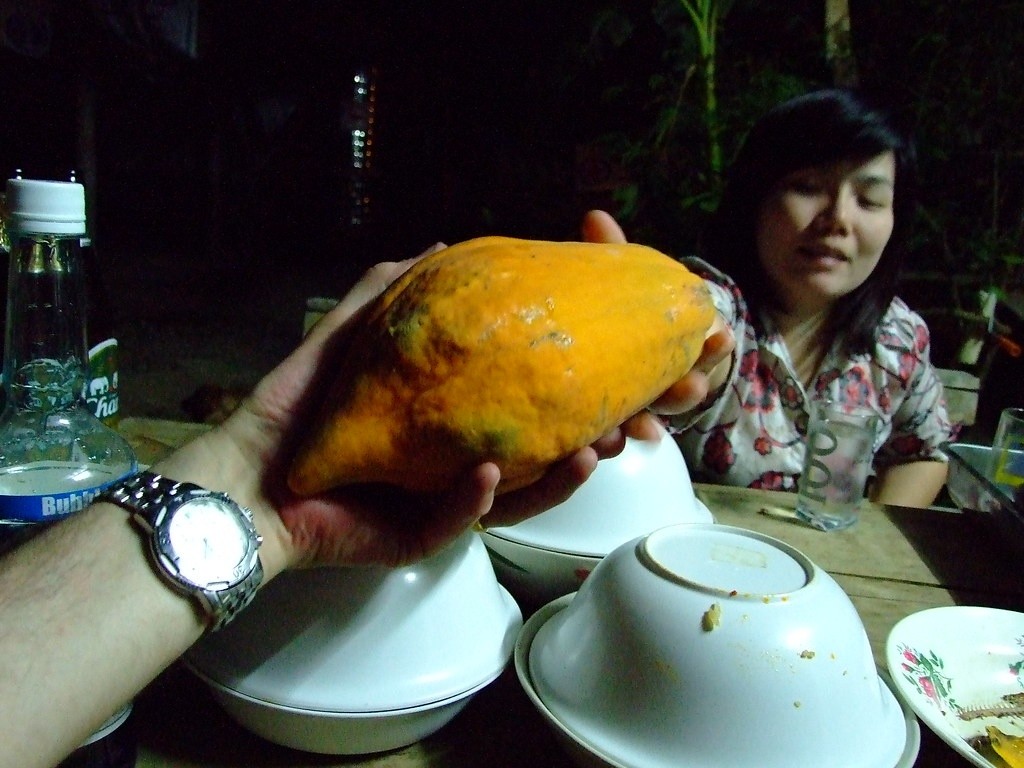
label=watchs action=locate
[91,472,264,636]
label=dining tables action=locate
[99,409,1023,768]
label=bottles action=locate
[0,178,133,768]
[299,67,382,339]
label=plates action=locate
[886,605,1024,768]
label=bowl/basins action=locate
[180,589,524,754]
[531,526,908,768]
[486,421,715,557]
[203,528,522,713]
[477,531,607,605]
[946,443,1024,514]
[513,524,919,768]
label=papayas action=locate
[288,239,714,498]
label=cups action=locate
[796,400,879,530]
[979,407,1024,511]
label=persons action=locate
[583,90,964,509]
[0,240,661,768]
[934,223,1001,372]
